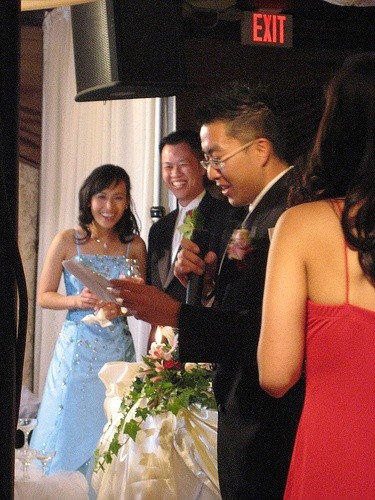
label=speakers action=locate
[71,0,198,102]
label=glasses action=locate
[201,139,264,171]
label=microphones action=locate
[186,228,210,305]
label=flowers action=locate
[92,326,219,474]
[177,208,208,241]
[227,230,252,261]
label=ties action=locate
[180,208,185,226]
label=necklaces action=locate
[88,224,118,250]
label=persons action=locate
[106,81,303,500]
[146,129,249,354]
[26,163,147,500]
[256,51,375,500]
